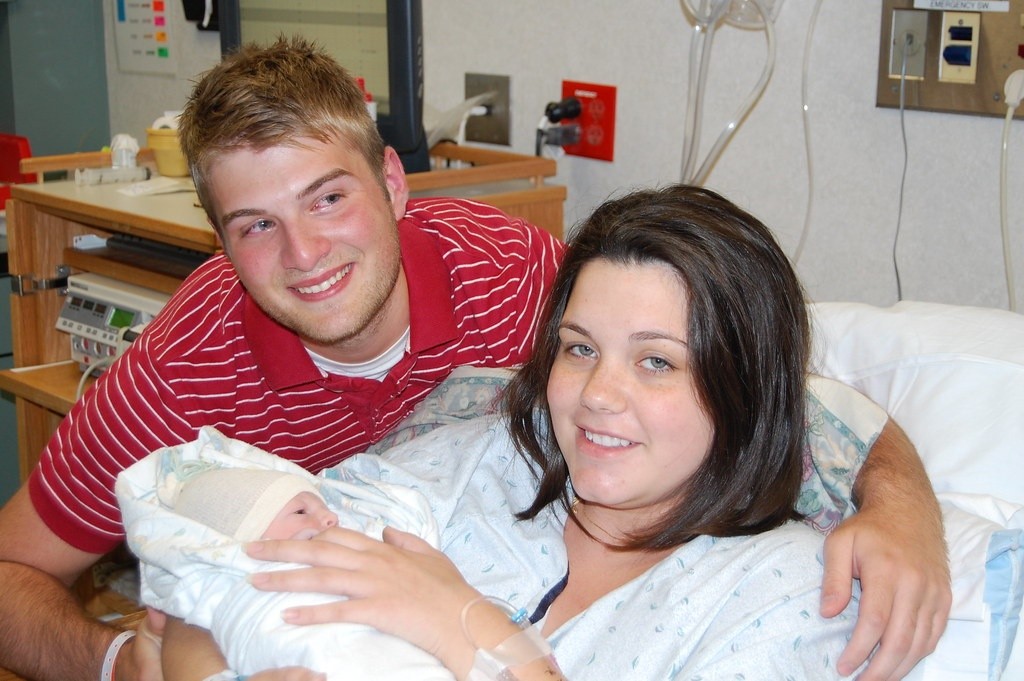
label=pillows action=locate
[366,367,1024,681]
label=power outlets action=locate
[875,0,1024,121]
[465,73,511,144]
[561,79,617,161]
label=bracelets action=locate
[100,630,137,681]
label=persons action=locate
[161,184,924,681]
[1,31,951,680]
[114,422,455,681]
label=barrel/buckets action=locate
[146,128,189,176]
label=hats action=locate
[156,449,327,551]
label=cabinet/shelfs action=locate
[0,141,568,488]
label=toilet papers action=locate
[150,114,184,131]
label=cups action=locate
[110,133,138,170]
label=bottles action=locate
[354,77,377,128]
